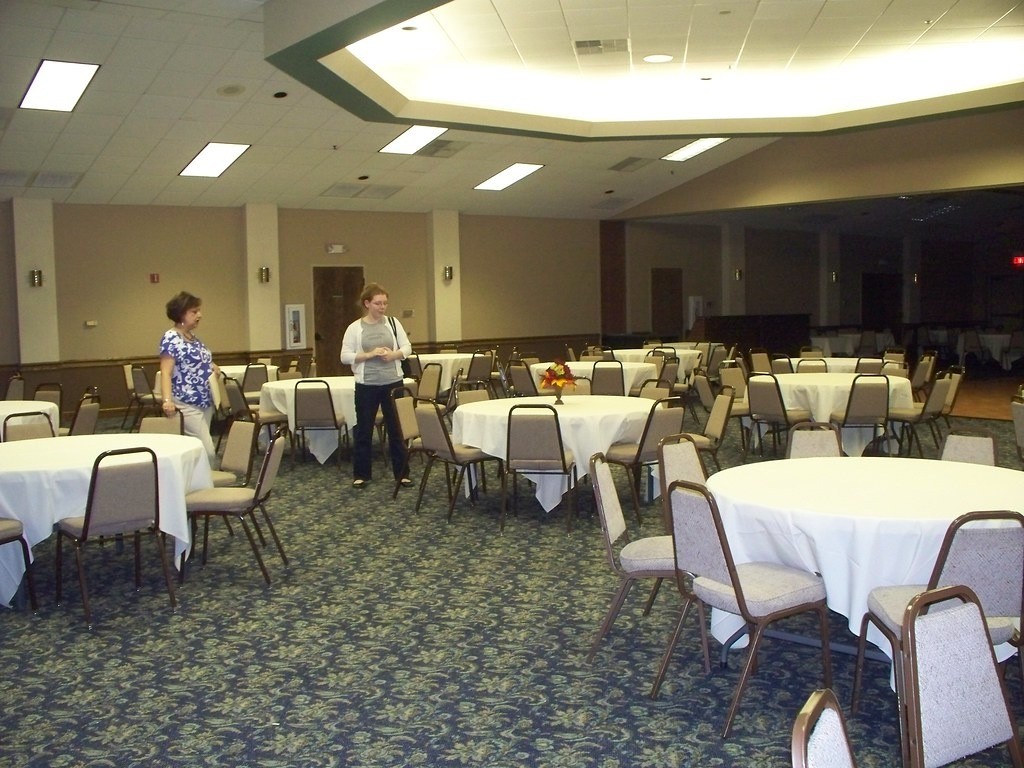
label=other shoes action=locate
[400,478,414,487]
[352,479,365,487]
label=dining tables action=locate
[528,360,658,395]
[644,341,726,364]
[212,364,279,390]
[775,357,899,375]
[407,352,485,390]
[2,433,203,588]
[604,347,702,384]
[702,455,1024,670]
[808,322,1017,371]
[453,394,663,513]
[0,399,60,441]
[744,371,913,457]
[260,376,416,465]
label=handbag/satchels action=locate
[388,317,411,378]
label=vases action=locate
[552,386,564,404]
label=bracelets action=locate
[161,398,171,402]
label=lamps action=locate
[735,268,742,281]
[260,265,271,283]
[914,273,919,284]
[31,268,42,287]
[326,243,349,255]
[832,271,838,282]
[444,265,453,280]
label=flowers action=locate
[538,360,579,390]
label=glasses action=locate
[372,302,388,305]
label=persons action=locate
[339,281,415,489]
[157,290,222,521]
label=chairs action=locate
[411,396,509,519]
[178,427,289,586]
[588,452,697,701]
[789,687,856,767]
[853,509,1024,716]
[500,404,580,535]
[905,583,1023,767]
[0,517,36,613]
[0,325,1024,479]
[188,409,266,549]
[650,478,832,737]
[53,447,176,623]
[644,434,710,615]
[590,396,685,528]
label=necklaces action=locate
[182,331,194,341]
